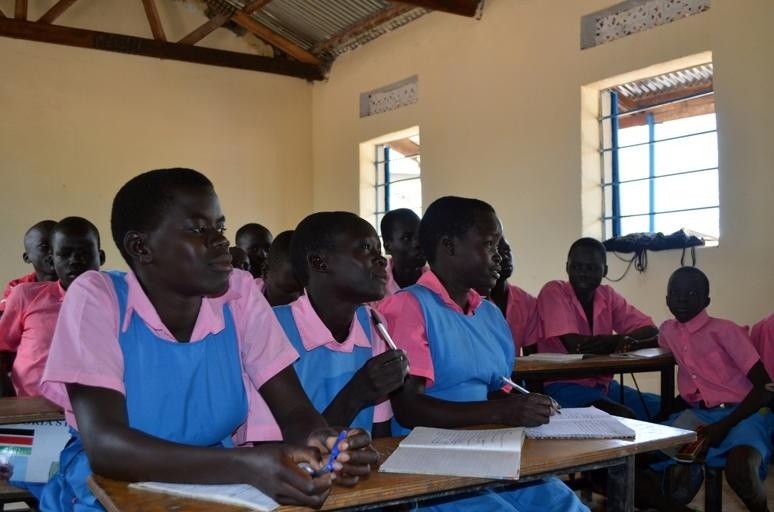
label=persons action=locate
[535,237,661,512]
[375,195,595,512]
[0,220,61,320]
[471,231,547,393]
[252,230,310,308]
[37,166,381,511]
[225,211,410,446]
[228,246,253,274]
[0,216,106,501]
[655,266,774,512]
[367,207,431,308]
[234,222,275,278]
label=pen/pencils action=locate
[325,430,347,472]
[499,376,562,414]
[370,308,410,372]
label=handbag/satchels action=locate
[602,230,703,269]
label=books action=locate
[378,426,528,482]
[525,404,636,440]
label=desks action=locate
[87,415,700,512]
[514,351,676,423]
[1,395,66,424]
